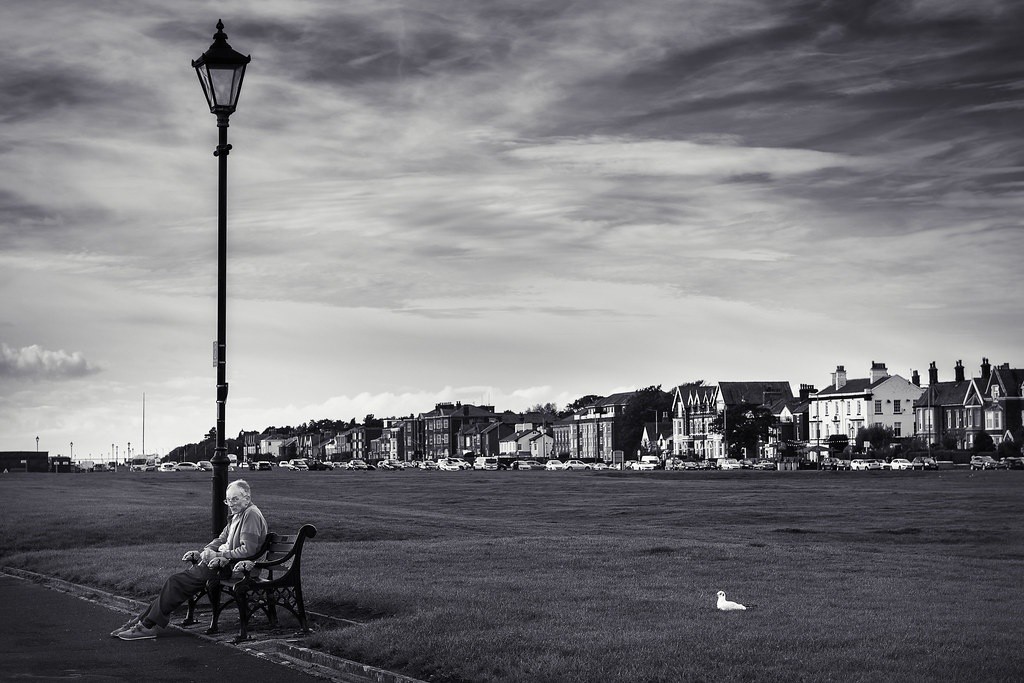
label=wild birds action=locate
[713,590,747,611]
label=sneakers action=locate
[110,618,157,640]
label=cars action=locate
[239,462,249,468]
[158,463,177,472]
[258,462,272,471]
[545,460,563,471]
[377,462,384,468]
[497,462,507,470]
[876,460,893,470]
[587,460,654,471]
[837,460,852,470]
[279,461,289,468]
[308,461,333,471]
[665,457,718,470]
[382,459,404,471]
[349,459,367,470]
[512,459,532,470]
[197,460,213,469]
[739,459,774,471]
[332,462,348,469]
[850,459,863,470]
[891,459,913,471]
[562,460,593,471]
[174,462,201,472]
[402,458,471,472]
[526,461,547,470]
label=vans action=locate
[473,457,498,471]
[642,455,661,469]
[717,459,740,471]
[130,455,156,473]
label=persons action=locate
[109,479,268,640]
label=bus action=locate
[226,454,237,467]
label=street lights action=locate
[191,16,251,541]
[36,436,39,452]
[70,442,73,461]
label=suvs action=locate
[970,456,999,471]
[911,457,938,471]
[289,459,310,471]
[857,460,881,471]
[821,457,841,470]
[997,458,1023,470]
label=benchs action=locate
[181,524,317,641]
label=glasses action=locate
[223,494,250,505]
